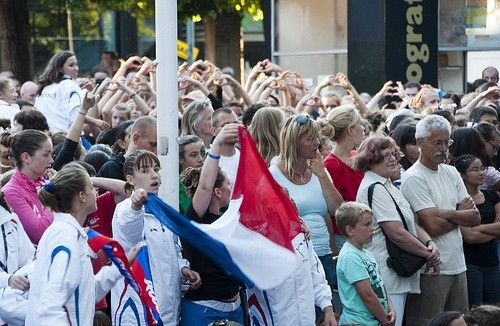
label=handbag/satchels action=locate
[385,234,427,277]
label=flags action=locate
[147,125,316,326]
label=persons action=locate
[0,261,37,326]
[316,105,364,255]
[263,187,338,326]
[111,150,202,326]
[351,135,442,326]
[0,52,500,238]
[335,202,397,326]
[428,305,500,326]
[266,113,343,318]
[25,167,146,326]
[0,130,57,326]
[180,124,253,326]
[400,115,481,326]
[455,154,500,307]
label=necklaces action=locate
[295,165,307,183]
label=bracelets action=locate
[79,112,86,115]
[130,92,135,98]
[426,240,431,246]
[209,153,220,159]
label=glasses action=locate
[422,138,454,148]
[466,167,488,173]
[296,115,310,126]
[382,152,398,160]
